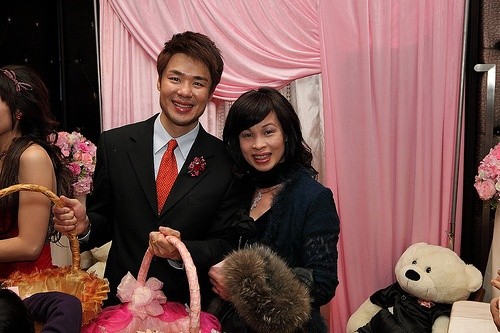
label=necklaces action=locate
[250,186,276,216]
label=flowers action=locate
[188,156,206,177]
[474,142,500,201]
[47,130,97,197]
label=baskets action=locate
[81,235,221,333]
[0,184,110,328]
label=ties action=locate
[155,140,179,217]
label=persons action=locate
[0,63,74,276]
[208,85,339,333]
[51,31,233,311]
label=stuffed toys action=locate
[345,241,483,333]
